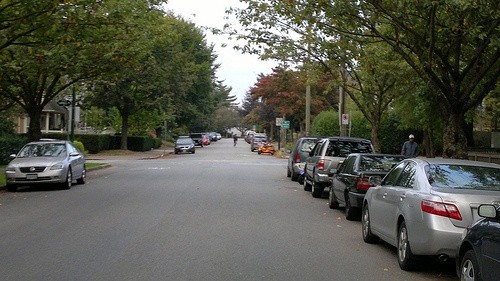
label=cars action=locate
[191,131,221,147]
[287,137,321,182]
[5,141,88,191]
[175,136,195,154]
[243,129,268,150]
[362,159,500,281]
[329,153,408,220]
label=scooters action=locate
[258,142,276,155]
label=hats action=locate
[409,134,414,138]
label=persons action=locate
[234,133,237,145]
[401,133,420,158]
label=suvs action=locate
[304,136,376,197]
